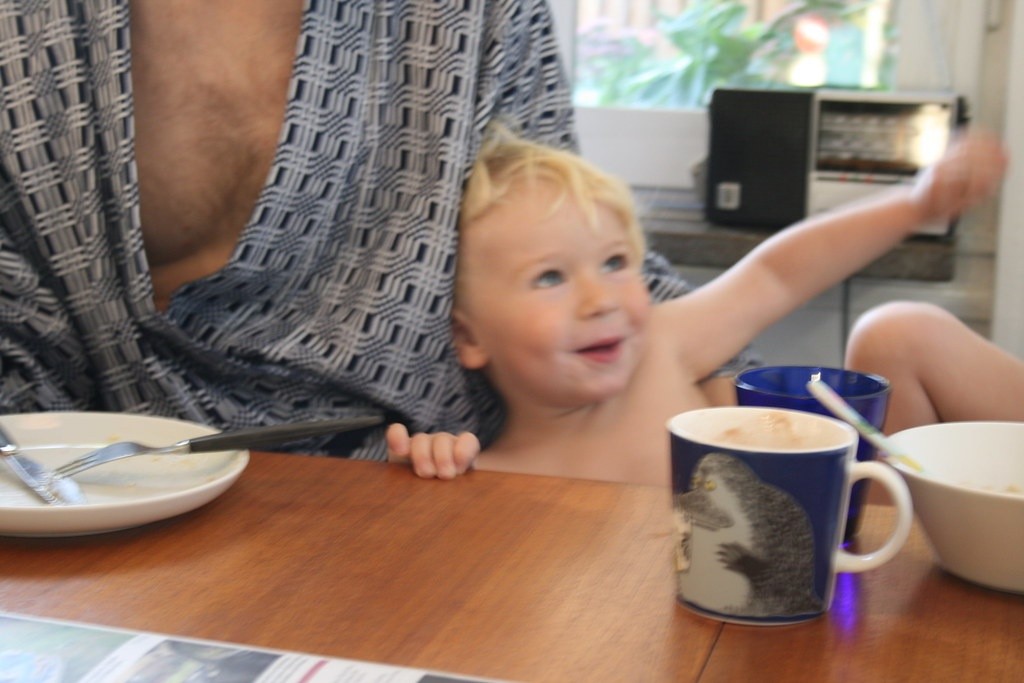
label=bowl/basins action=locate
[879,422,1024,594]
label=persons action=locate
[0,0,751,462]
[386,120,1024,504]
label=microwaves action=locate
[704,87,971,240]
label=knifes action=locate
[0,427,66,506]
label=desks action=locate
[0,447,1024,683]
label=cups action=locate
[666,406,914,626]
[733,366,894,543]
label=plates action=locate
[0,412,250,537]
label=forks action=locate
[50,414,386,482]
